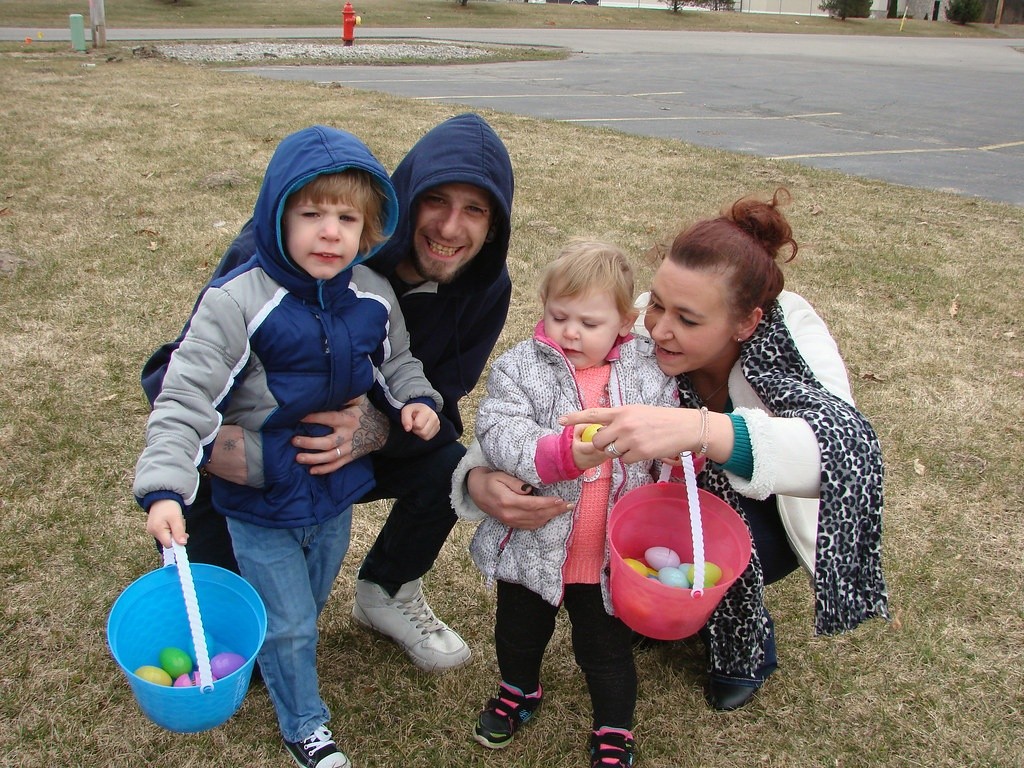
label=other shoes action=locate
[709,679,755,711]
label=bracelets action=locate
[678,408,705,458]
[695,405,711,460]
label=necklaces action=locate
[702,379,727,404]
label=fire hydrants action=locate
[342,2,361,46]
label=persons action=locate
[129,123,446,765]
[468,240,707,768]
[449,200,887,713]
[139,113,516,673]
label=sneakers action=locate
[351,568,473,674]
[283,724,350,768]
[473,680,543,748]
[591,727,637,768]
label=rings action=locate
[336,447,341,459]
[607,442,623,456]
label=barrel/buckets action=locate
[608,450,752,641]
[106,536,267,733]
